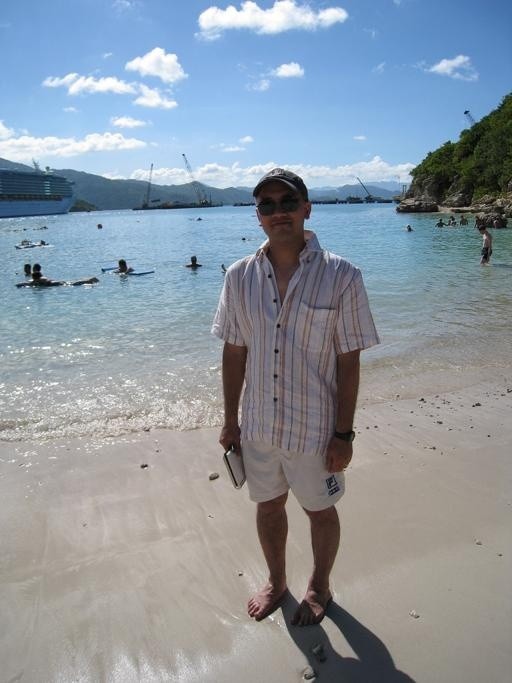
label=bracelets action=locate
[333,428,356,441]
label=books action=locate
[223,447,247,489]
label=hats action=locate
[252,167,309,201]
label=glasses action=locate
[256,198,300,215]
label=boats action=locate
[0,162,76,217]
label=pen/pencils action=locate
[231,443,234,452]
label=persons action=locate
[448,216,453,225]
[33,263,42,272]
[450,217,456,226]
[97,223,103,229]
[460,214,468,226]
[478,225,492,265]
[24,263,32,276]
[15,270,100,287]
[185,255,202,267]
[472,215,507,228]
[113,258,135,276]
[209,167,383,628]
[406,224,414,231]
[434,218,448,227]
